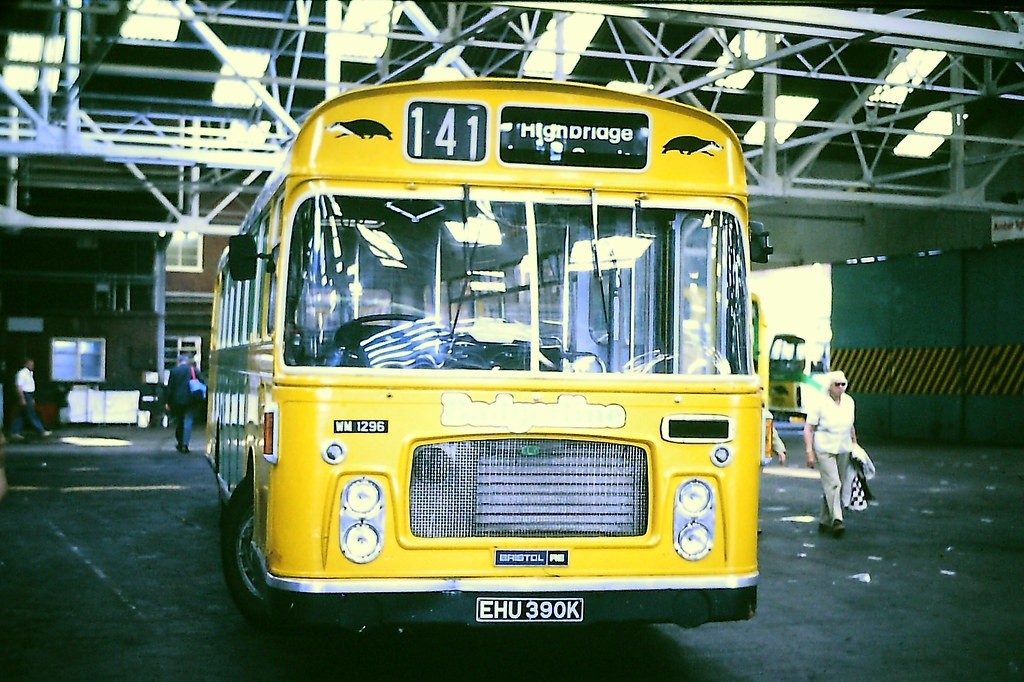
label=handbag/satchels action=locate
[846,456,870,512]
[188,365,209,401]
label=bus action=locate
[769,331,824,418]
[201,74,758,636]
[748,293,775,467]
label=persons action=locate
[804,370,857,537]
[164,354,206,453]
[757,385,787,536]
[9,355,53,441]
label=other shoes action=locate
[39,429,53,437]
[817,520,834,531]
[8,433,25,441]
[831,518,846,537]
[175,442,189,453]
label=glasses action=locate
[832,382,847,388]
